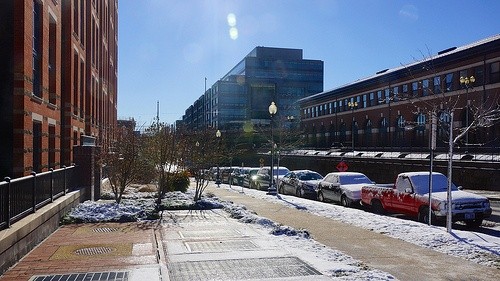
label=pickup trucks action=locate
[362,171,491,227]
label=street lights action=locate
[348,102,358,151]
[216,130,221,184]
[460,76,476,143]
[379,93,403,146]
[269,102,276,190]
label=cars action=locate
[201,167,323,200]
[320,172,375,207]
